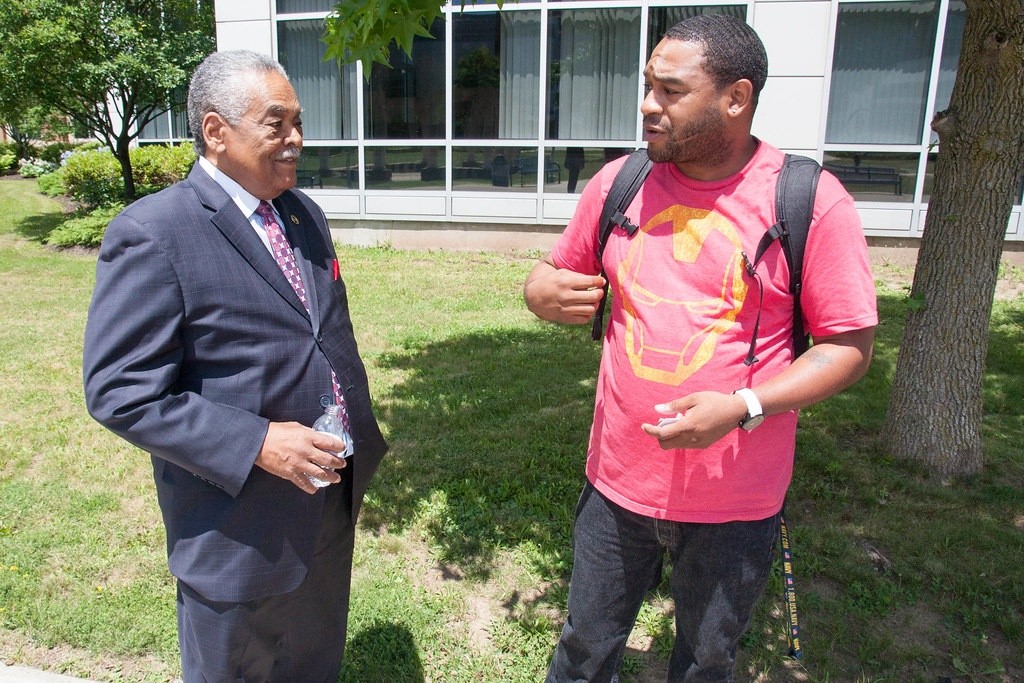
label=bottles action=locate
[303,406,342,488]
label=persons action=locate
[564,147,587,193]
[84,49,388,682]
[524,14,881,683]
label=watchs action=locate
[731,387,765,433]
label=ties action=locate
[255,199,355,433]
